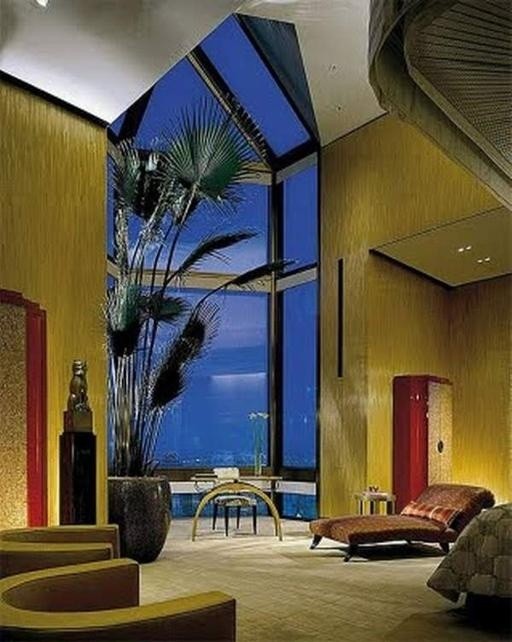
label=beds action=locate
[427,504,512,626]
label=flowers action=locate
[248,412,268,477]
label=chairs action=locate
[0,558,237,642]
[0,524,121,582]
[309,485,496,562]
[212,467,257,536]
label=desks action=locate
[190,475,284,542]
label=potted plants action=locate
[101,89,299,565]
